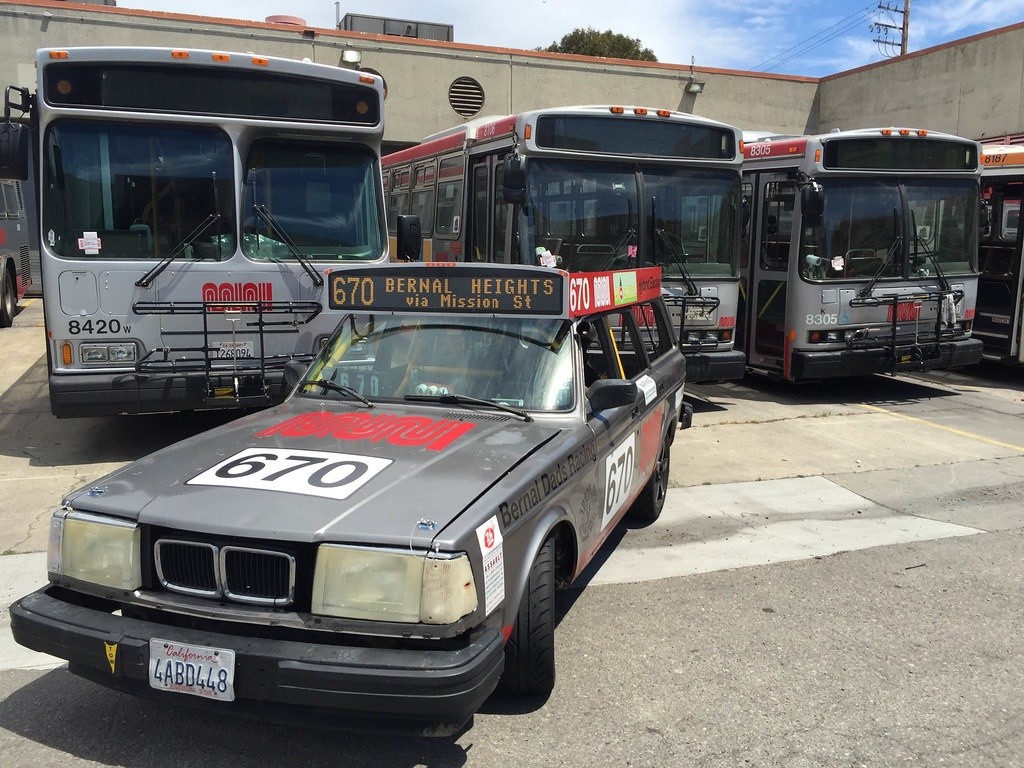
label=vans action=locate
[0,180,32,329]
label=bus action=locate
[682,127,990,385]
[368,105,748,381]
[0,48,420,419]
[907,144,1024,365]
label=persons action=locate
[133,134,228,254]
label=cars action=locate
[8,262,687,742]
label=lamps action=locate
[341,42,362,64]
[43,12,53,19]
[303,29,316,38]
[685,80,705,94]
